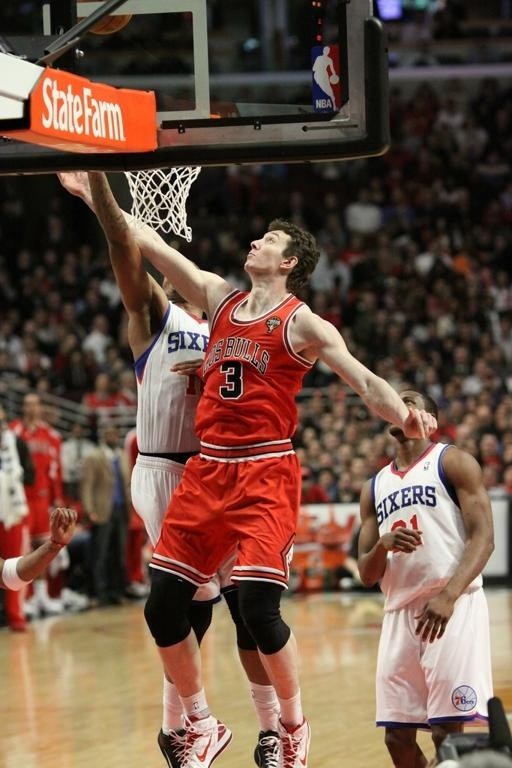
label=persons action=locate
[336,524,382,593]
[1,0,511,503]
[56,171,441,768]
[54,171,292,768]
[2,392,151,632]
[356,390,496,768]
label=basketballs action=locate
[76,15,132,35]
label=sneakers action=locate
[254,728,280,768]
[158,727,188,768]
[23,582,151,620]
[277,715,311,768]
[180,713,232,768]
